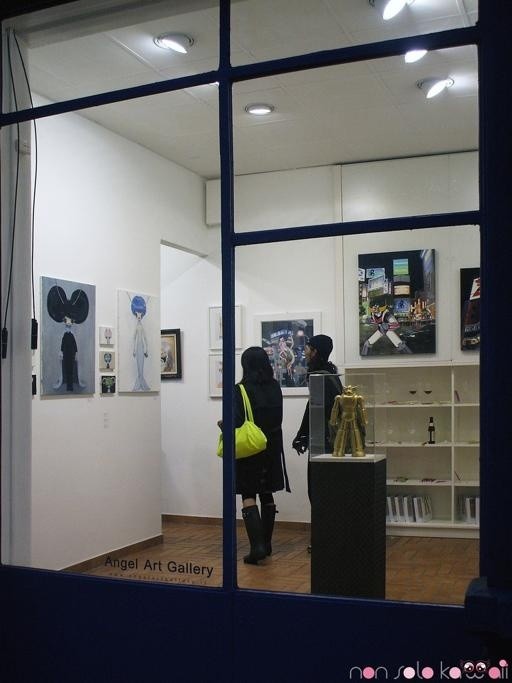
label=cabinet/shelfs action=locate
[343,363,480,540]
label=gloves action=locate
[292,435,309,456]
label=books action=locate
[386,493,433,523]
[465,497,480,525]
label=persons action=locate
[292,334,366,553]
[328,384,369,456]
[217,346,291,563]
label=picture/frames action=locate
[251,311,323,396]
[161,328,182,379]
[207,353,241,399]
[208,305,243,351]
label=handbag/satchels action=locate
[216,383,268,459]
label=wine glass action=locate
[407,384,416,405]
[423,383,432,403]
[407,421,417,444]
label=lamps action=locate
[368,0,413,21]
[152,29,194,56]
[414,76,454,99]
[244,101,275,117]
[404,48,428,64]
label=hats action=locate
[307,335,333,358]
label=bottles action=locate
[427,416,435,444]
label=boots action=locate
[261,504,276,555]
[241,505,265,562]
